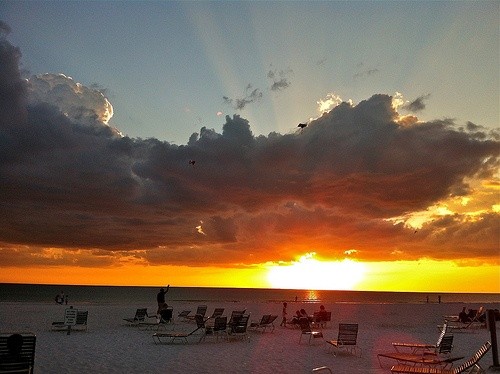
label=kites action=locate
[189,160,195,166]
[297,123,306,135]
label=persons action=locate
[280,303,287,327]
[438,295,441,303]
[287,309,308,324]
[157,285,169,315]
[55,291,68,304]
[457,307,471,323]
[320,305,327,324]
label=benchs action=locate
[0,333,36,374]
[51,311,88,332]
[123,305,493,374]
